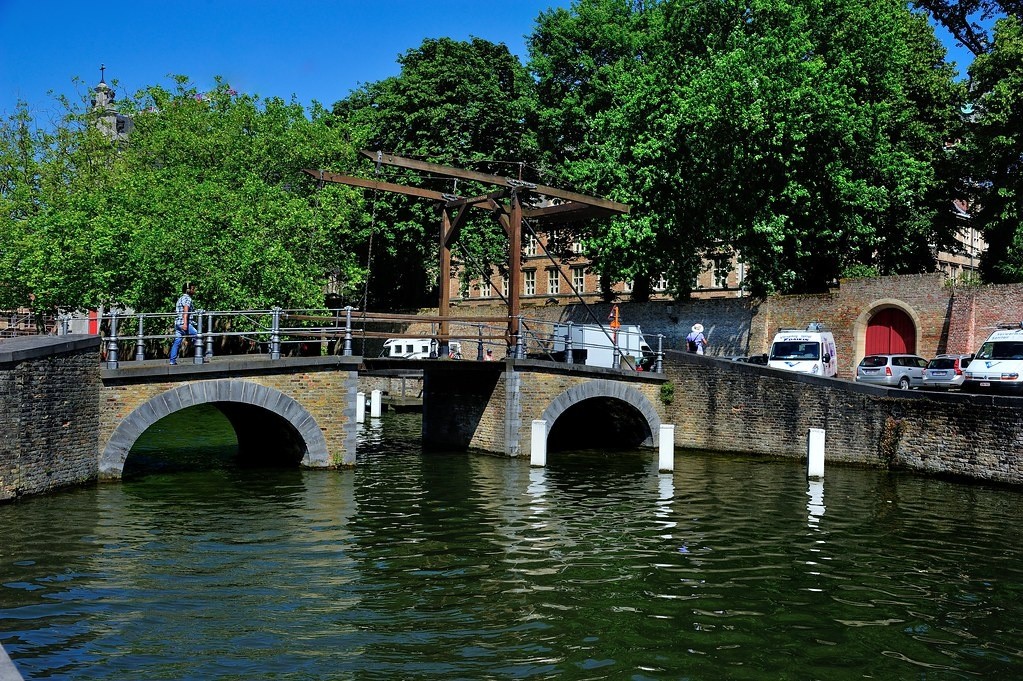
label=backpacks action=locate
[689,333,702,351]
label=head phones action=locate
[186,283,190,293]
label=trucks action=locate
[552,322,656,371]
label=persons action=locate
[685,324,707,355]
[484,348,495,361]
[170,283,202,365]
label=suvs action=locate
[855,353,928,390]
[920,353,977,394]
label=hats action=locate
[692,324,704,332]
[487,349,492,353]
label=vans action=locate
[767,326,839,379]
[377,339,461,360]
[966,322,1023,396]
